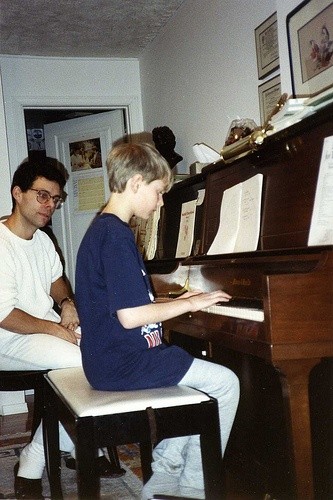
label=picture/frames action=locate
[257,74,281,127]
[255,11,280,80]
[285,0,333,98]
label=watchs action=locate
[57,297,74,307]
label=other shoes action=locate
[14,461,45,500]
[141,472,179,500]
[66,455,126,478]
[177,485,206,500]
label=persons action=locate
[75,142,241,500]
[0,155,127,500]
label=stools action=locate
[0,370,48,440]
[41,367,223,500]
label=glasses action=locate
[28,188,65,209]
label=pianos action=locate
[144,101,333,500]
